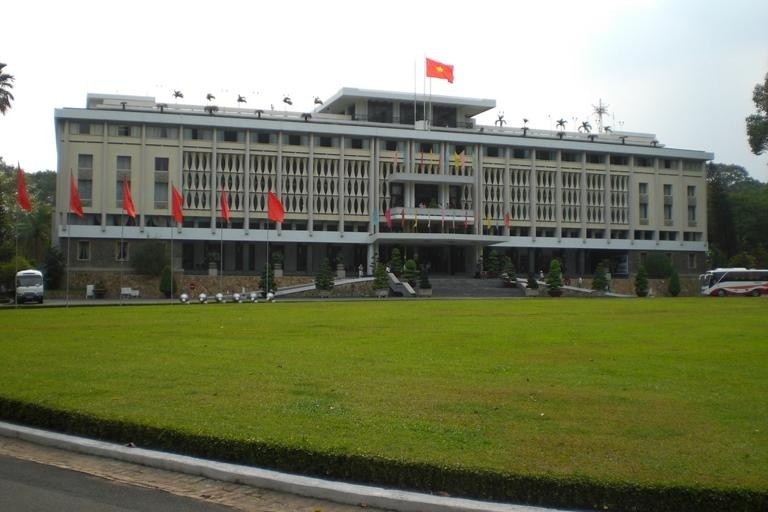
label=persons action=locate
[577,274,584,289]
[385,265,390,272]
[442,121,448,128]
[538,269,545,282]
[358,263,364,277]
[419,199,426,209]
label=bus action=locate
[698,267,768,297]
[15,269,44,305]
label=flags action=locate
[504,211,510,226]
[426,207,431,228]
[487,214,492,230]
[373,205,380,227]
[460,150,465,170]
[14,164,32,215]
[428,148,433,170]
[413,208,418,228]
[385,208,392,227]
[420,148,424,170]
[425,56,455,84]
[122,175,138,219]
[454,151,458,171]
[440,206,445,228]
[399,209,405,229]
[451,211,456,229]
[171,183,184,225]
[463,212,468,229]
[68,170,84,218]
[268,192,285,224]
[221,189,231,224]
[495,214,499,230]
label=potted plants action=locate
[314,256,337,298]
[92,280,108,299]
[257,263,277,299]
[372,261,390,298]
[415,263,433,298]
[485,248,682,297]
[159,265,179,299]
